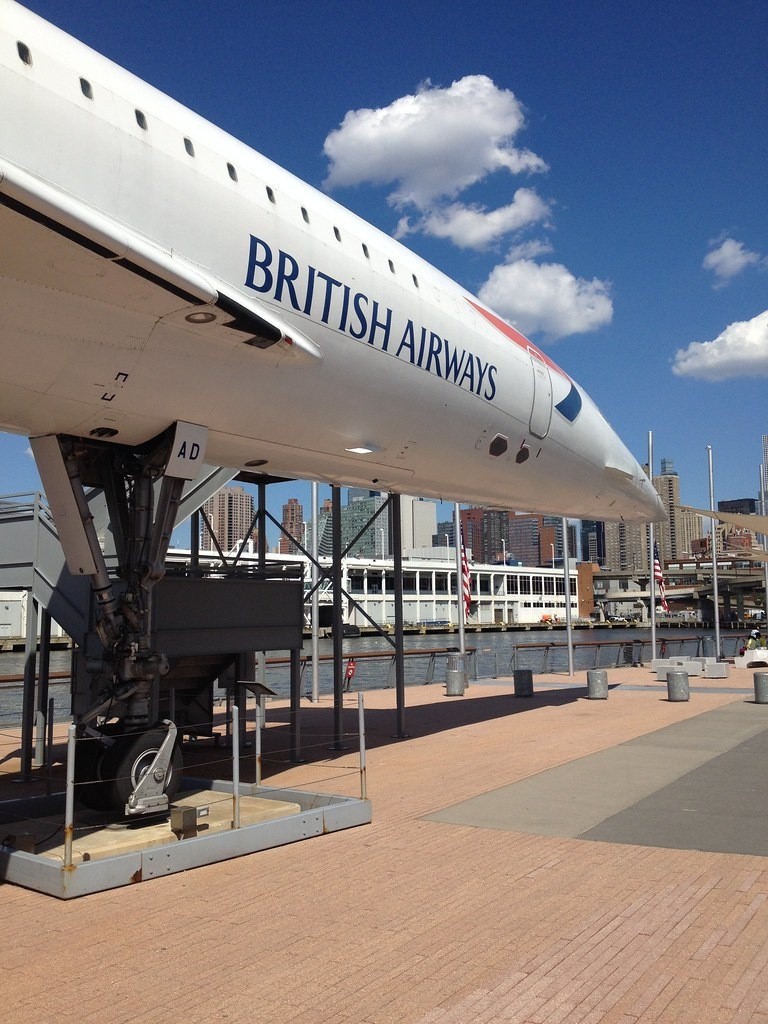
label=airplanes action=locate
[0,0,672,523]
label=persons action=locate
[745,628,762,650]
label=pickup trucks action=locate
[610,615,625,622]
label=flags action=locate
[460,519,472,618]
[653,537,671,613]
